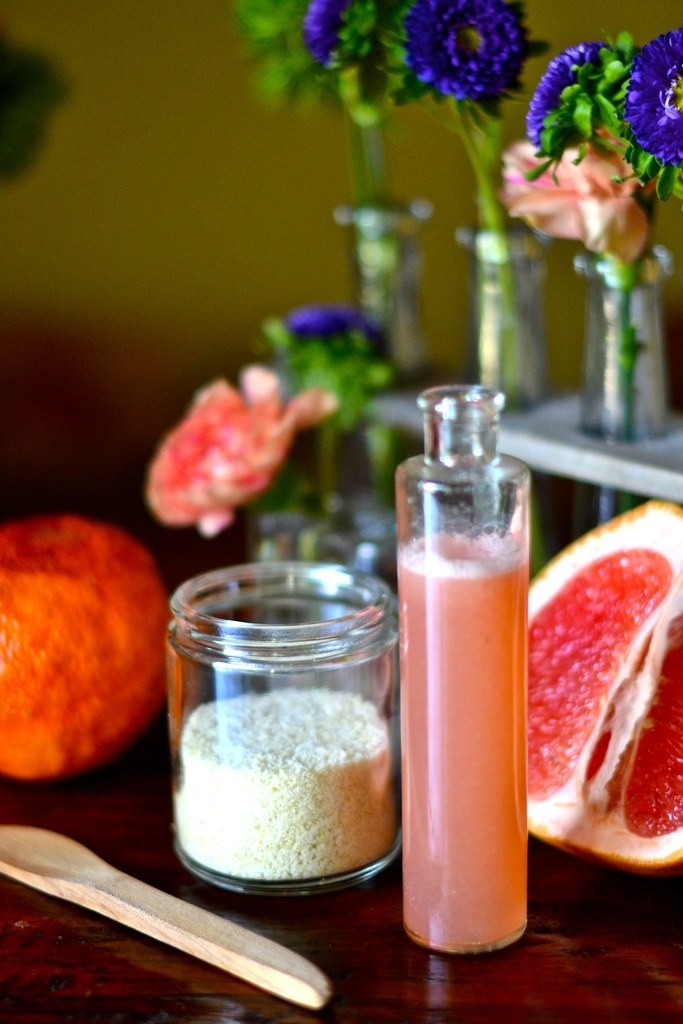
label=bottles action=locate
[393,384,531,956]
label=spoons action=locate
[1,824,333,1011]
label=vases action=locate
[572,249,669,443]
[253,496,404,580]
[470,223,546,409]
[336,199,430,388]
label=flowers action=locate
[143,299,401,542]
[379,1,553,224]
[503,26,683,247]
[234,1,417,200]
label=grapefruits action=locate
[0,513,170,787]
[475,498,683,885]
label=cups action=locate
[165,562,403,897]
